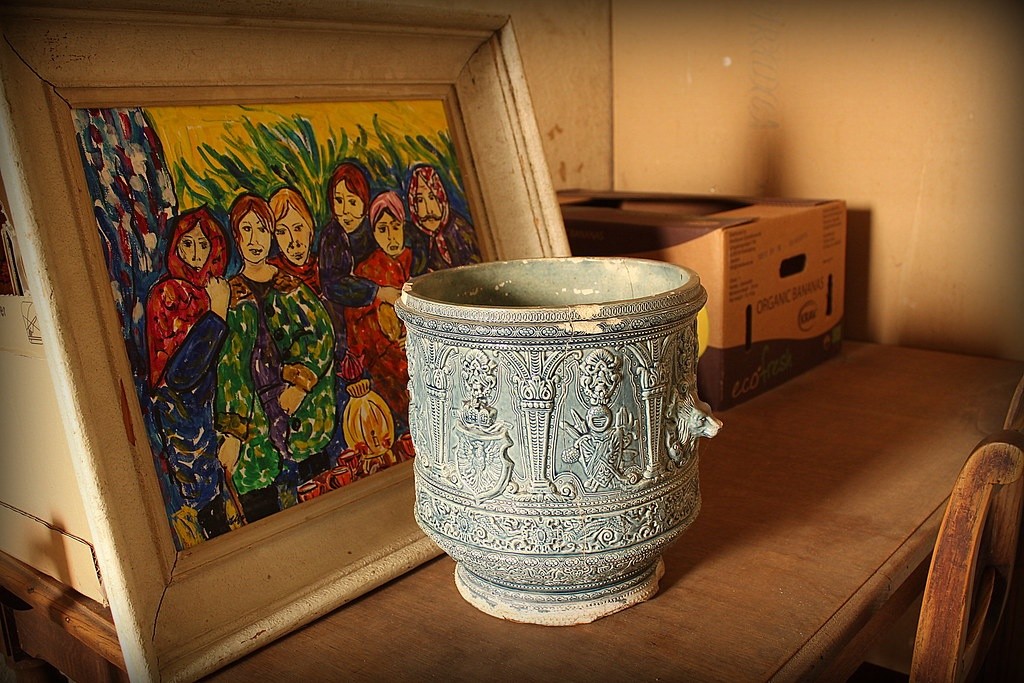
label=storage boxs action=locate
[547,186,850,413]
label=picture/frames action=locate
[0,3,576,683]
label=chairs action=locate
[851,431,1024,682]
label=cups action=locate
[392,256,724,627]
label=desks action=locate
[191,343,1023,683]
